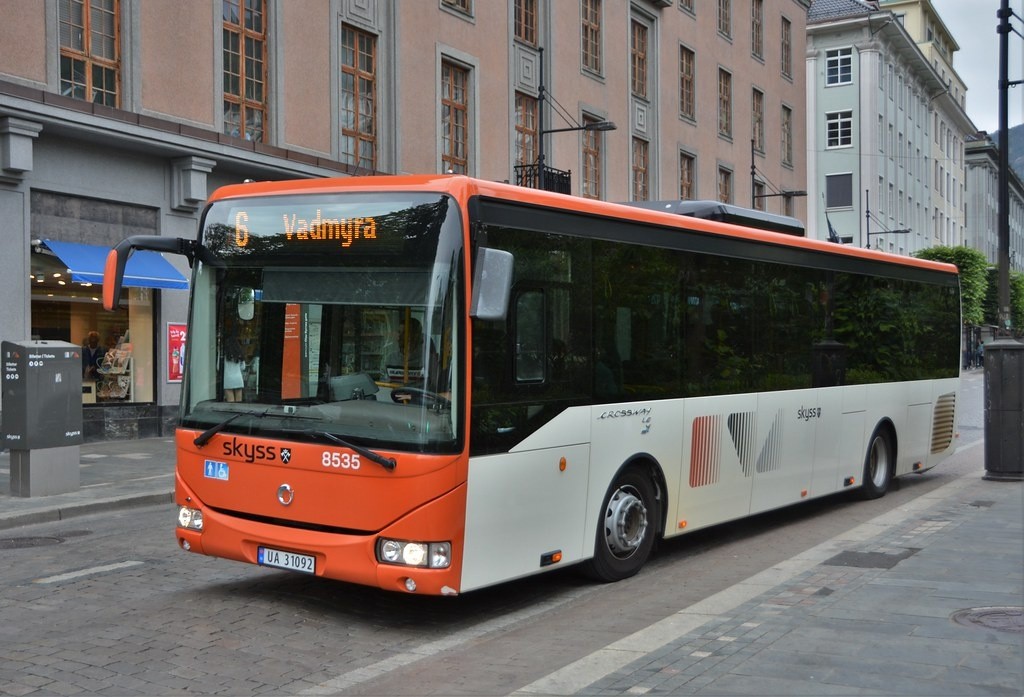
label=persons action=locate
[222,336,247,402]
[975,339,984,368]
[79,330,109,381]
[380,317,438,401]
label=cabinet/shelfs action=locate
[95,357,134,403]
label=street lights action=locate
[865,188,911,249]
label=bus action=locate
[100,171,963,599]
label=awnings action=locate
[43,241,189,290]
